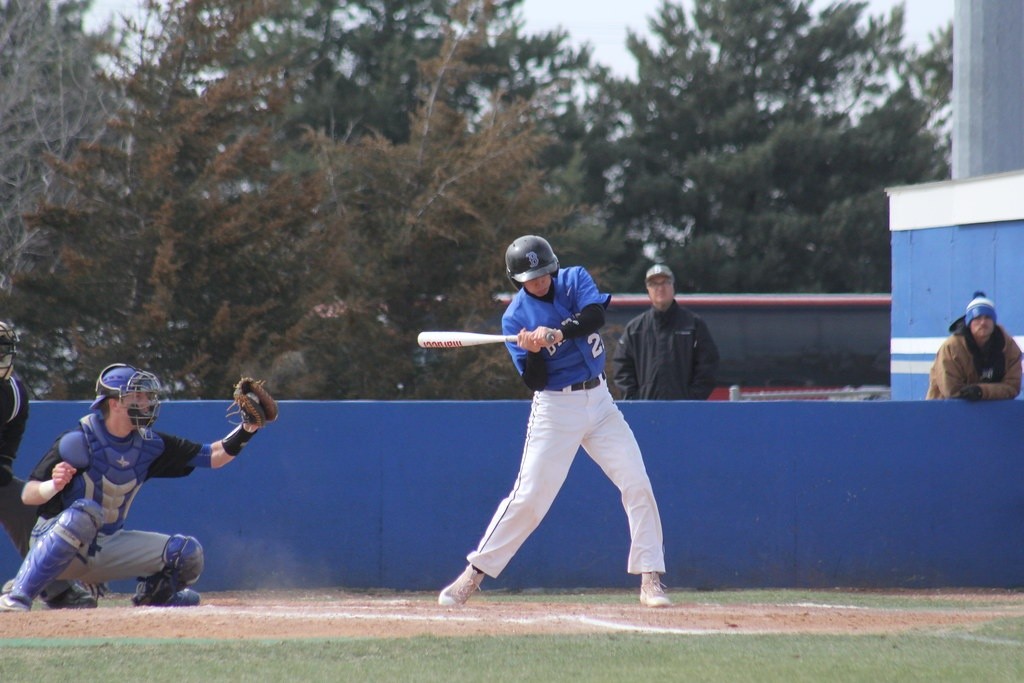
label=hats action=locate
[646,264,673,278]
[965,291,997,326]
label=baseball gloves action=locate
[233,377,279,427]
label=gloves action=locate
[962,386,981,400]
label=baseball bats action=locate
[416,331,556,349]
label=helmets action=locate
[90,363,159,410]
[0,321,17,381]
[504,235,558,282]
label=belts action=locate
[557,372,605,391]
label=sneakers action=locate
[439,562,485,609]
[1,580,35,611]
[45,583,97,609]
[133,585,201,606]
[639,573,674,605]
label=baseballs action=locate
[246,392,261,405]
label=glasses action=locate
[648,280,674,288]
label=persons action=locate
[0,320,107,609]
[0,361,279,610]
[439,236,671,607]
[610,264,720,401]
[925,291,1023,400]
